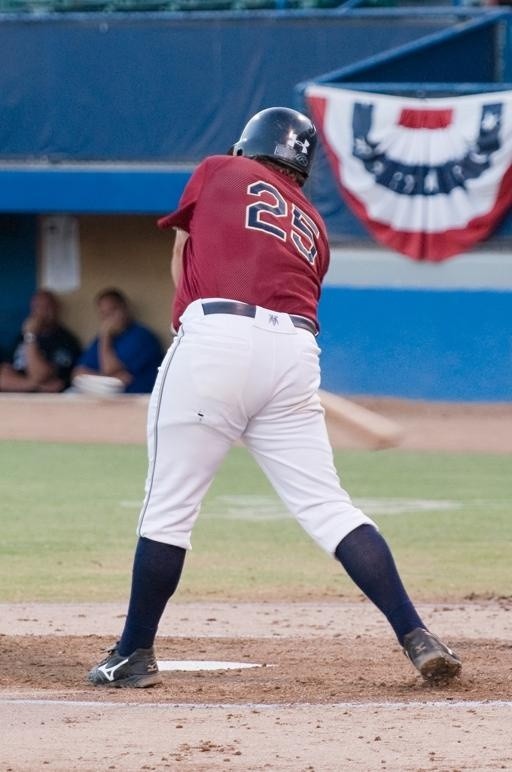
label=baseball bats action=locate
[319,390,405,450]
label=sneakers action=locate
[89,641,162,688]
[402,627,463,679]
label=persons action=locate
[0,291,81,392]
[88,107,462,688]
[72,292,167,392]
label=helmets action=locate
[228,107,318,178]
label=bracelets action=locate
[24,333,38,341]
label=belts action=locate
[202,302,317,335]
